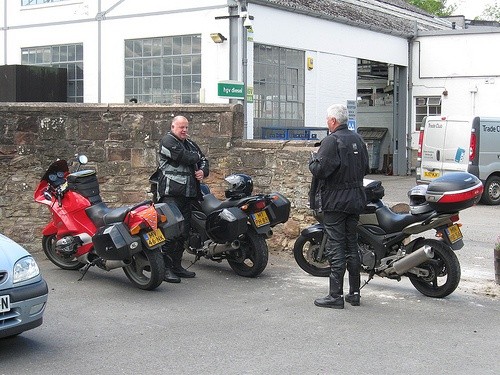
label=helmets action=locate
[407,185,436,214]
[224,174,253,199]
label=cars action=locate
[0,233,48,339]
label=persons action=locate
[158,116,210,283]
[309,103,370,309]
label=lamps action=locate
[210,34,228,43]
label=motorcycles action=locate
[147,159,291,278]
[34,154,186,289]
[294,142,484,297]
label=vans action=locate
[415,116,500,206]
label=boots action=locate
[345,274,360,306]
[161,250,180,282]
[314,274,344,309]
[171,248,196,277]
